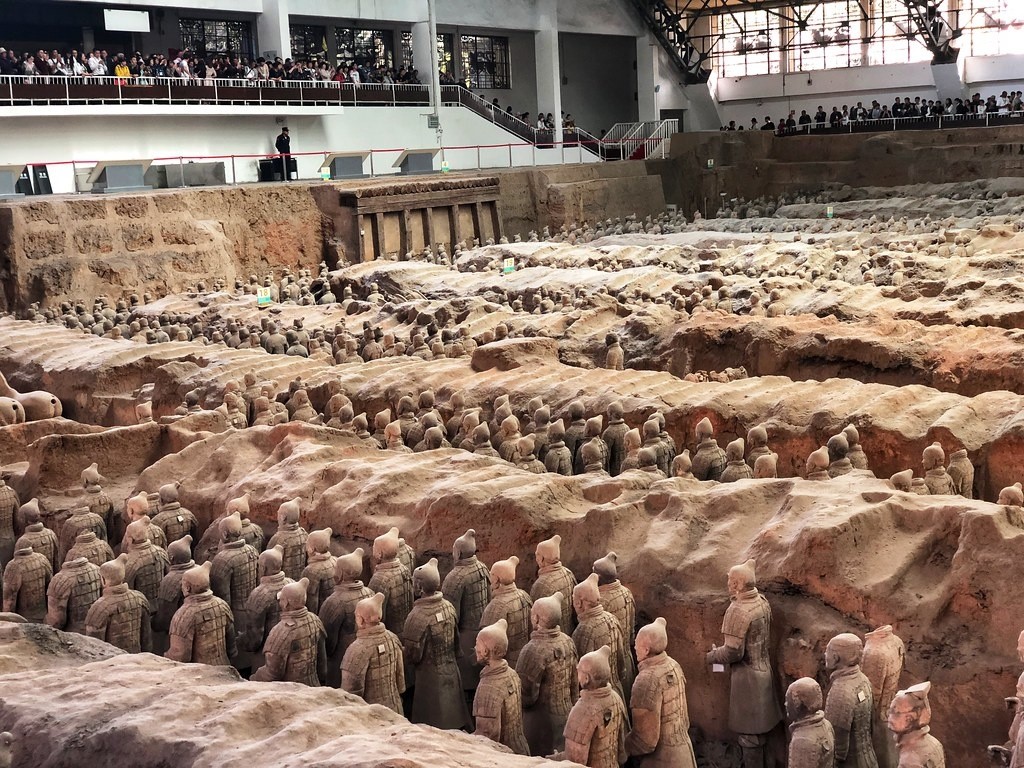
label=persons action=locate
[275,127,294,181]
[0,186,1024,768]
[0,47,422,105]
[437,69,1024,134]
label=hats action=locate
[282,127,289,131]
[750,118,758,123]
[0,47,7,53]
